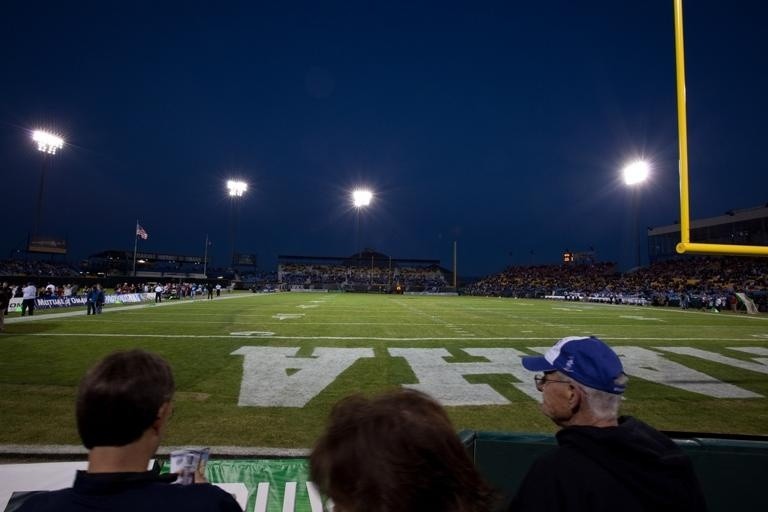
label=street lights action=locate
[351,188,374,265]
[227,179,249,267]
[28,129,68,240]
[624,158,655,270]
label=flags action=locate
[136,224,148,240]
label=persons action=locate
[1,345,246,512]
[279,261,449,294]
[502,332,714,512]
[0,259,279,317]
[304,385,512,512]
[460,253,768,317]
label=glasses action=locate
[535,374,571,392]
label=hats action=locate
[523,336,626,394]
